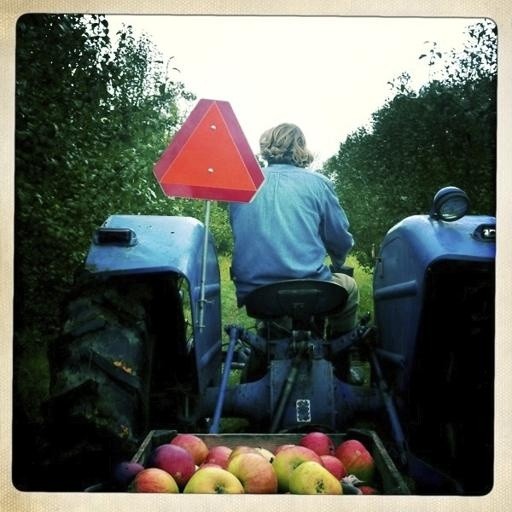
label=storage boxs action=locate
[119,429,413,495]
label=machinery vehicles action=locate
[48,186,494,494]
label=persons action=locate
[226,122,366,387]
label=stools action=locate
[245,281,349,340]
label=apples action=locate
[111,432,378,494]
